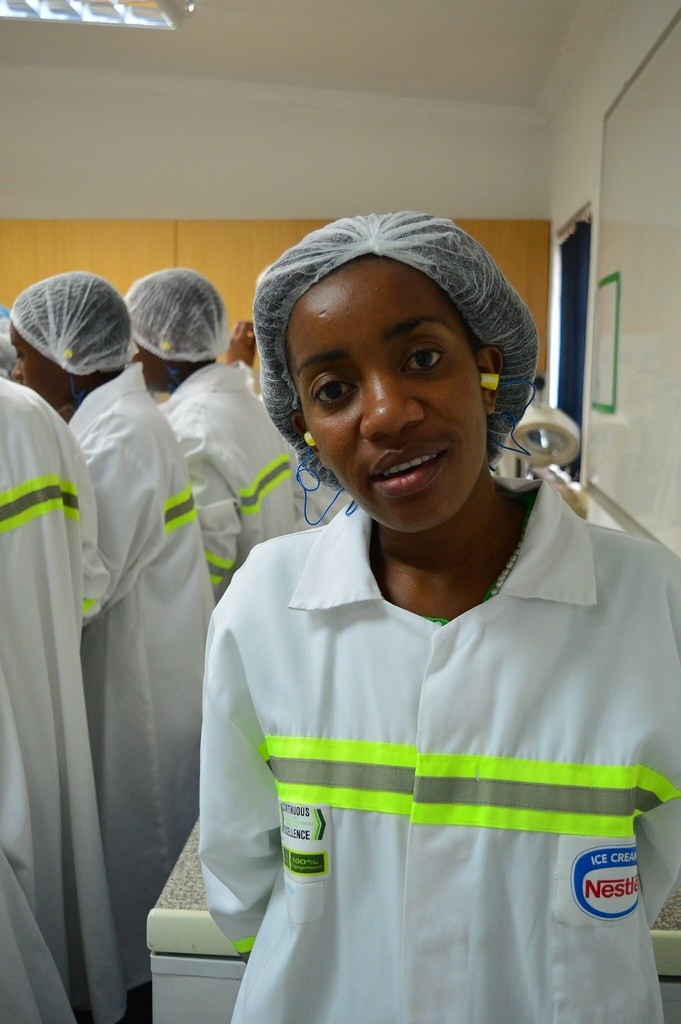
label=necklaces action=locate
[426,505,527,629]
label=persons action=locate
[122,265,305,608]
[198,212,681,1024]
[222,315,353,532]
[1,376,131,1024]
[8,271,215,1024]
